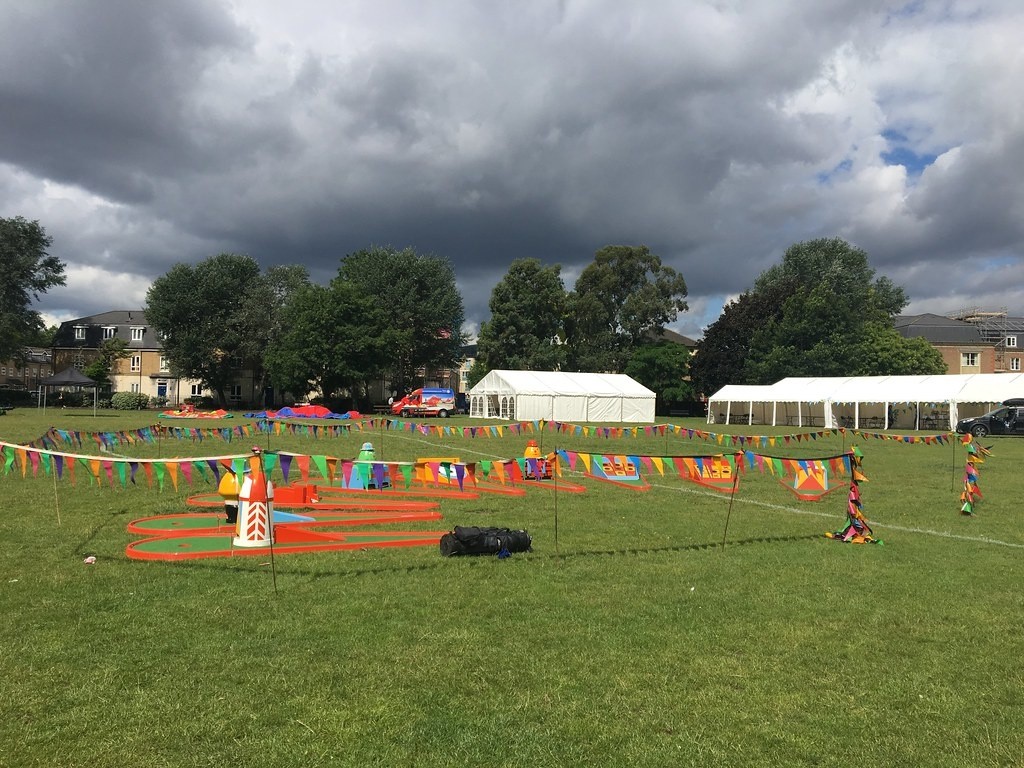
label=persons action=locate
[385,395,393,416]
[58,390,66,409]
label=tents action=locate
[707,373,1024,432]
[37,366,99,417]
[469,369,656,423]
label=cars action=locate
[956,406,1024,438]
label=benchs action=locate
[718,413,755,426]
[843,417,885,429]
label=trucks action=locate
[392,388,456,419]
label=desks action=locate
[785,415,824,427]
[920,418,951,431]
